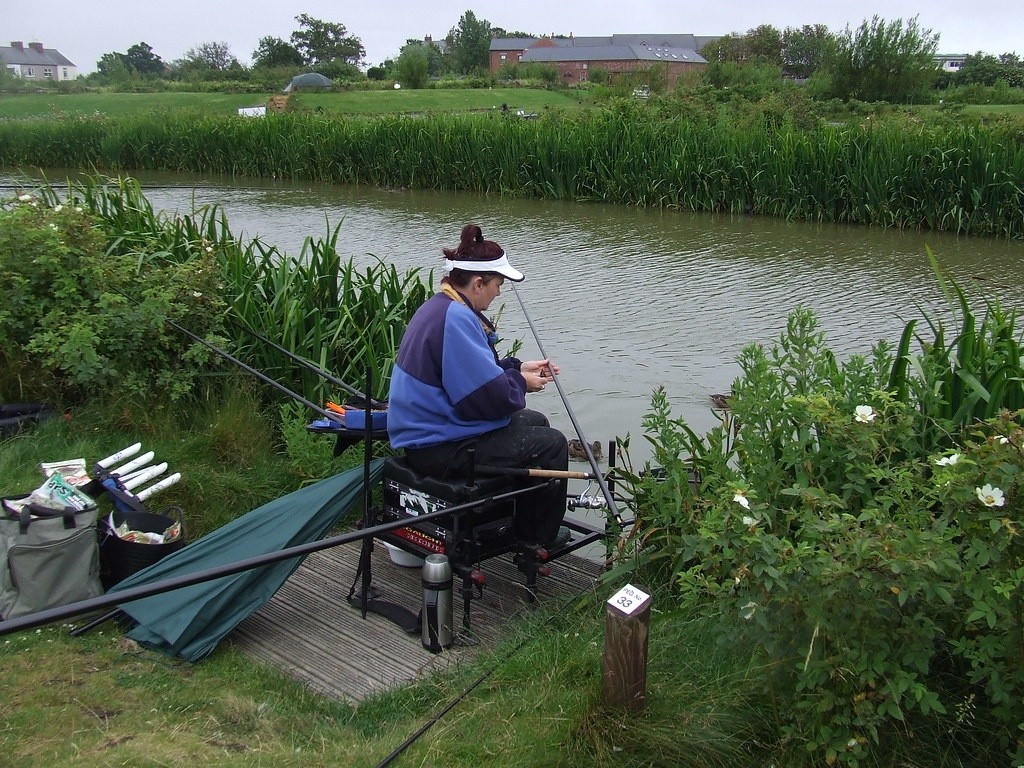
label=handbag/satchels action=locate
[97,505,186,594]
[0,493,105,624]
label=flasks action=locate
[419,553,456,652]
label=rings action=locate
[542,385,545,390]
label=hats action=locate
[441,249,525,282]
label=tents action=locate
[283,73,337,94]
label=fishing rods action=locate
[0,185,320,190]
[1,247,623,768]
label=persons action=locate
[387,223,572,561]
[501,103,511,113]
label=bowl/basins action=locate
[386,543,425,570]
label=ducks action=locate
[569,439,603,461]
[709,394,731,409]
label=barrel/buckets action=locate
[97,505,184,595]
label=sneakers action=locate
[546,525,571,551]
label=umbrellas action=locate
[102,456,389,669]
